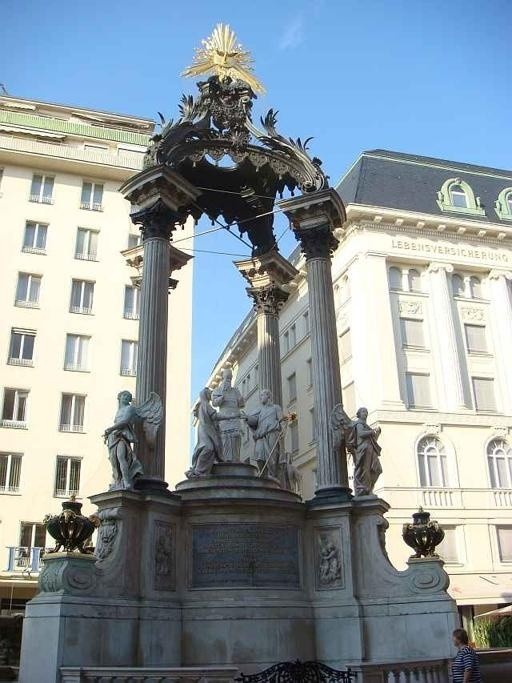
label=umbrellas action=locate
[473,603,512,619]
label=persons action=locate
[351,407,381,497]
[191,368,303,496]
[103,391,142,490]
[449,629,482,683]
